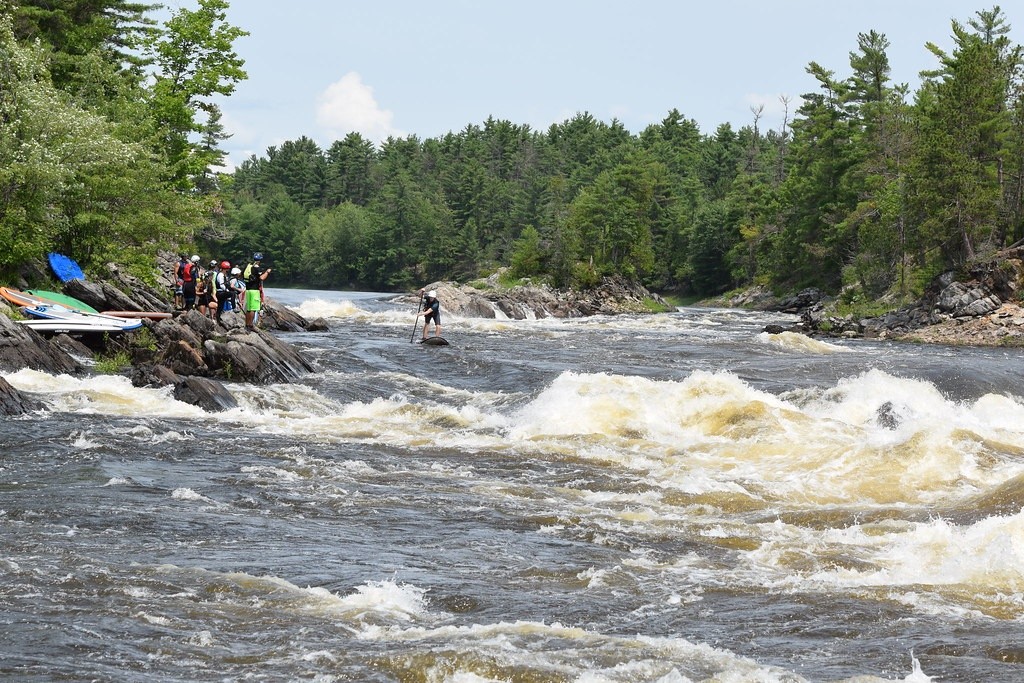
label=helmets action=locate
[254,252,263,261]
[181,252,188,257]
[231,268,241,275]
[221,261,231,269]
[191,255,200,263]
[211,260,218,265]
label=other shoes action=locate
[245,323,259,333]
[231,308,240,313]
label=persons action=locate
[174,252,243,322]
[416,288,441,340]
[244,252,272,333]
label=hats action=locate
[428,290,436,298]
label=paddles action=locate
[203,284,209,304]
[237,301,246,315]
[410,290,423,343]
[261,302,290,331]
